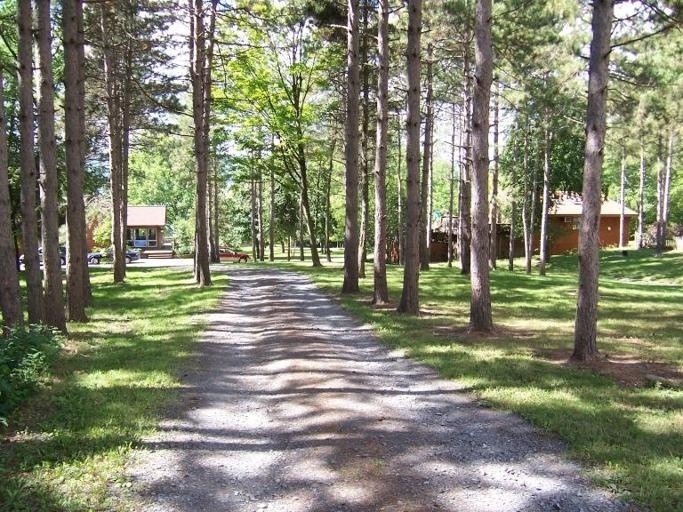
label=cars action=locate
[219,247,249,264]
[18,245,66,266]
[87,246,140,265]
